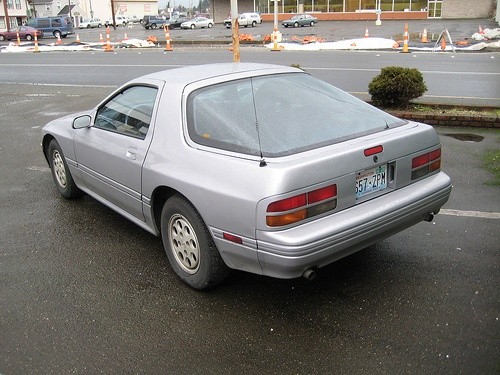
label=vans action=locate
[24,15,76,39]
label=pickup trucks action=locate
[223,12,262,29]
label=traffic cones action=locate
[98,32,104,42]
[123,32,129,40]
[421,28,429,43]
[478,26,484,34]
[56,35,63,45]
[75,33,81,42]
[363,27,369,38]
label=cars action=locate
[281,14,318,28]
[104,14,188,29]
[0,25,43,41]
[180,16,215,29]
[39,61,452,291]
[77,18,103,29]
